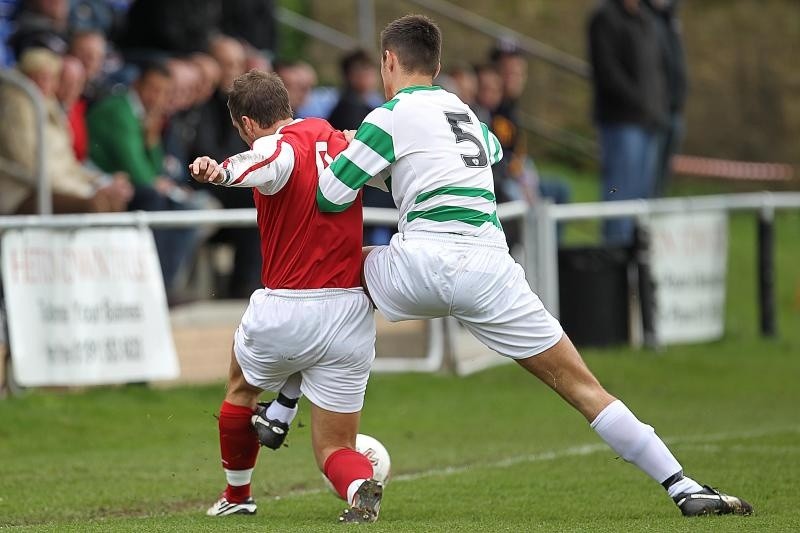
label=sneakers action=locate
[206,497,258,517]
[671,484,755,518]
[337,478,384,524]
[251,401,290,451]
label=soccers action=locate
[321,432,392,500]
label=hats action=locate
[490,32,523,60]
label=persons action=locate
[0,0,573,310]
[584,0,676,302]
[187,69,388,526]
[211,14,753,516]
[639,0,686,199]
